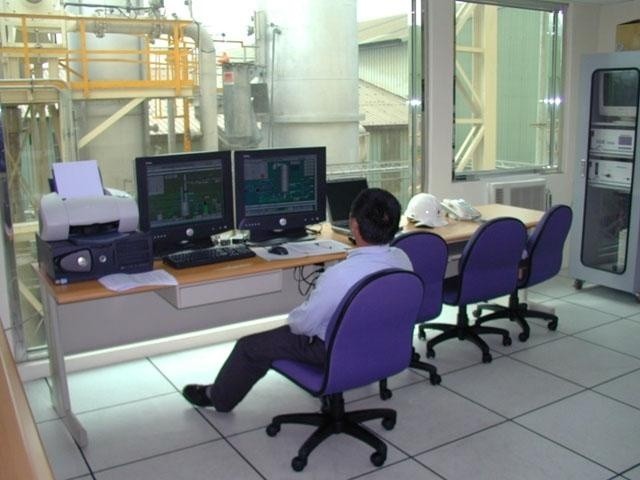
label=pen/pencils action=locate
[315,243,331,249]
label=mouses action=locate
[268,246,289,256]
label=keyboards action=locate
[163,244,256,269]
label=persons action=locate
[182,187,415,413]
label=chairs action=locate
[265,268,425,472]
[473,204,573,342]
[379,231,448,400]
[418,217,528,364]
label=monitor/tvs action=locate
[135,150,234,247]
[235,145,327,242]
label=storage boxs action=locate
[616,18,640,50]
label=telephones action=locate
[440,198,482,221]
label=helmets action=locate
[404,193,448,227]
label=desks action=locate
[31,204,546,448]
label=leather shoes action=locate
[182,384,215,407]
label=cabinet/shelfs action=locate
[572,51,640,303]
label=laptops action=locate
[328,177,403,235]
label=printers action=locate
[35,167,153,285]
